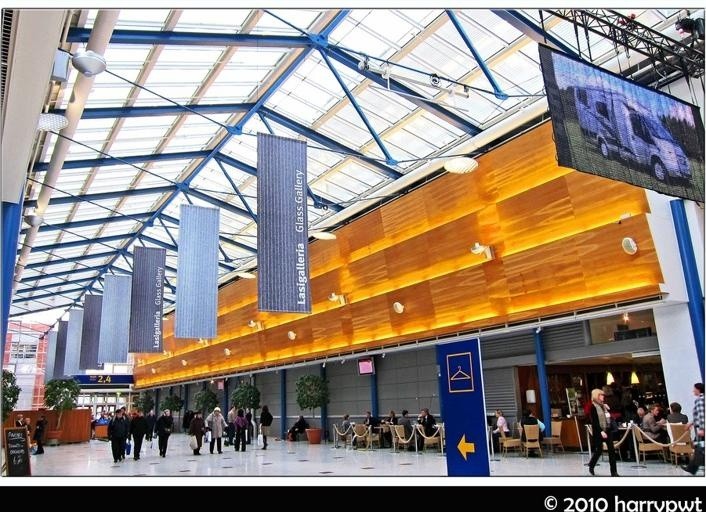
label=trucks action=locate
[567,76,695,189]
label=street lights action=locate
[115,391,122,408]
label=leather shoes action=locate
[681,464,697,474]
[589,463,596,475]
[611,472,620,476]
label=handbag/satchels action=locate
[165,428,171,434]
[257,434,264,448]
[692,435,705,466]
[224,440,229,446]
[150,435,160,450]
[125,439,131,455]
[189,435,198,450]
[204,427,212,443]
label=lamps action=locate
[237,155,483,282]
[22,47,107,227]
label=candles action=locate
[295,373,328,444]
[44,377,82,439]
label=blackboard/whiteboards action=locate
[4,427,31,476]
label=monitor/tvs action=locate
[216,379,224,391]
[356,357,375,376]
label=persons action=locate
[492,408,510,452]
[515,408,545,454]
[609,386,692,462]
[584,388,621,476]
[342,413,357,445]
[21,417,33,450]
[363,411,376,433]
[679,382,705,474]
[91,401,274,464]
[15,414,25,426]
[31,416,48,455]
[393,410,415,452]
[417,408,439,450]
[381,409,397,447]
[288,414,305,441]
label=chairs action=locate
[332,400,692,463]
